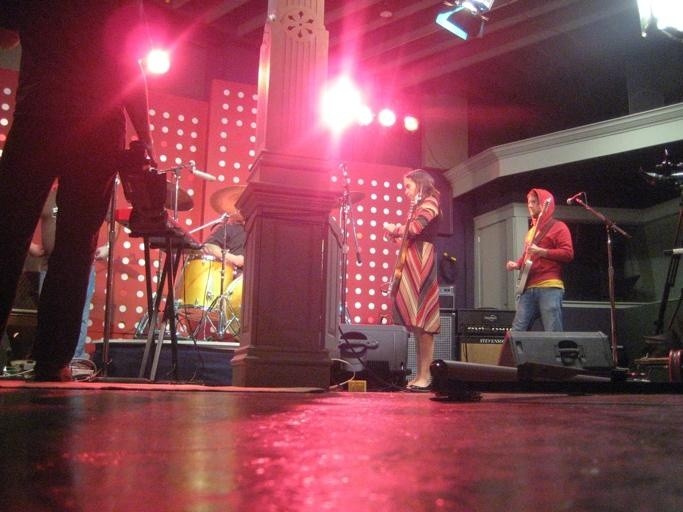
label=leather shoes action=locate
[411,382,434,392]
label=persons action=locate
[1,0,157,382]
[203,210,248,278]
[383,169,442,390]
[506,188,575,366]
[29,191,119,379]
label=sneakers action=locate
[33,362,76,381]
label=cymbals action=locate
[165,179,194,212]
[210,187,244,222]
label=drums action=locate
[222,275,243,339]
[175,251,237,316]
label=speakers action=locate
[497,331,614,369]
[339,324,409,386]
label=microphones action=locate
[354,245,363,263]
[567,193,582,206]
[192,165,216,181]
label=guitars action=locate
[513,200,551,300]
[388,192,420,298]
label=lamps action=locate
[433,1,493,40]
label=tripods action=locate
[133,210,240,340]
